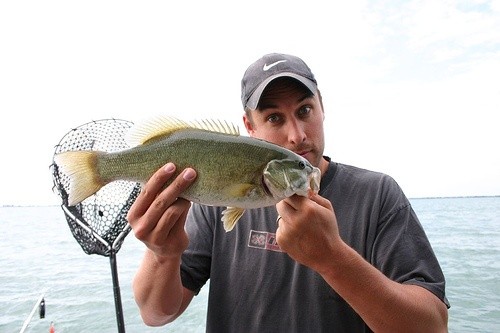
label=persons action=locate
[126,53,451,333]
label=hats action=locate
[241,52,317,111]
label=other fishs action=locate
[52,115,323,234]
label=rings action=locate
[276,216,282,225]
